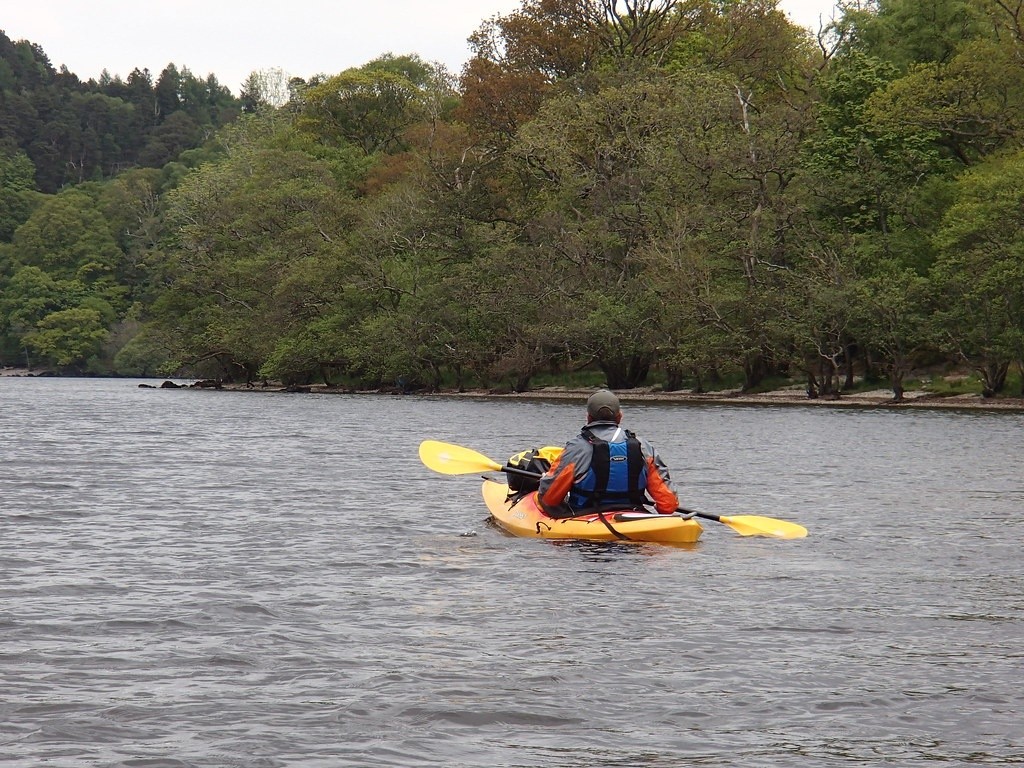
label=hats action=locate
[587,389,621,421]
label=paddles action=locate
[418,438,810,541]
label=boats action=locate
[481,477,704,542]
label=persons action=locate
[538,389,680,514]
